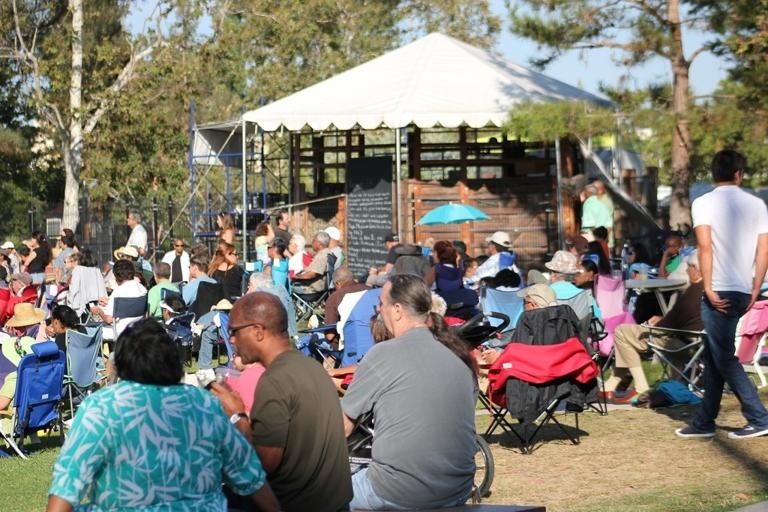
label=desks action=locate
[478,284,537,332]
[621,278,686,319]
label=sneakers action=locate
[675,418,718,438]
[191,328,203,338]
[194,368,218,388]
[728,421,768,440]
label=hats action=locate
[319,226,342,242]
[4,302,48,328]
[1,242,15,251]
[486,230,512,248]
[381,233,400,243]
[114,245,139,262]
[388,255,428,279]
[515,284,557,308]
[564,236,589,252]
[1,335,40,369]
[159,299,187,316]
[544,250,584,275]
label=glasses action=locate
[627,250,636,256]
[175,244,184,248]
[227,323,266,336]
[231,252,239,256]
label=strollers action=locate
[296,310,510,497]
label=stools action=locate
[351,505,546,512]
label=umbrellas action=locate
[413,201,492,228]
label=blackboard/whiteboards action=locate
[345,156,393,281]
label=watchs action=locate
[229,411,249,425]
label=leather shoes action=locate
[612,388,641,404]
[596,386,618,404]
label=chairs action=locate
[477,305,608,454]
[292,253,337,323]
[297,323,336,366]
[214,313,234,366]
[0,340,67,460]
[160,312,195,373]
[730,300,768,389]
[641,298,708,408]
[106,294,148,350]
[450,311,509,353]
[59,324,108,420]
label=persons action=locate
[341,272,479,512]
[578,184,614,236]
[44,317,283,512]
[208,290,355,512]
[588,179,616,260]
[674,146,768,440]
[1,209,764,452]
